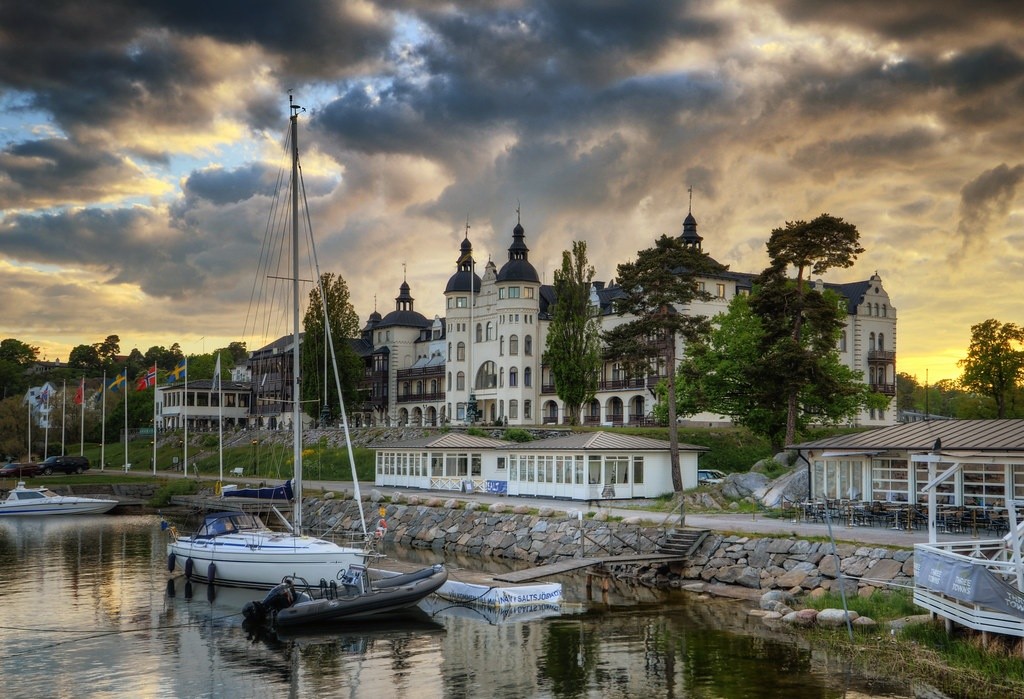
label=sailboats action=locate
[160,88,385,588]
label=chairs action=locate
[783,500,1024,537]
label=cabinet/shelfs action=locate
[639,411,668,427]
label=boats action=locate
[0,469,119,516]
[244,564,448,625]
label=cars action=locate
[698,470,726,480]
[0,464,41,478]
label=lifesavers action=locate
[376,519,387,538]
[215,481,221,496]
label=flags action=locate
[136,365,156,391]
[94,381,103,401]
[29,386,48,408]
[211,353,220,392]
[72,380,82,404]
[106,371,125,391]
[168,359,185,383]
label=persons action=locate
[373,526,384,540]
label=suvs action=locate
[37,456,91,476]
